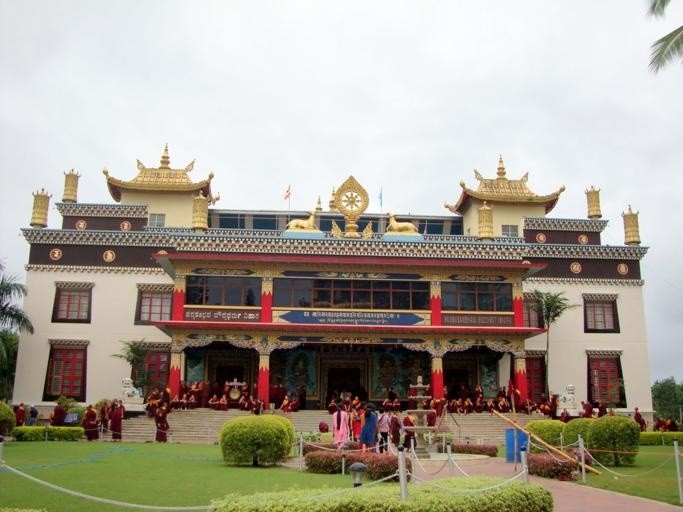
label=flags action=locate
[378,191,383,207]
[284,188,290,199]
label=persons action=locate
[80,399,125,443]
[143,379,306,417]
[423,383,522,417]
[427,408,436,426]
[319,384,418,451]
[15,401,67,426]
[525,392,678,432]
[155,410,170,444]
